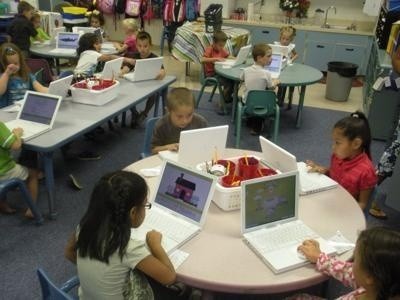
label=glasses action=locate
[138,202,151,209]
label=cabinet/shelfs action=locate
[251,26,305,63]
[305,31,373,75]
[363,36,380,116]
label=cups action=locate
[215,159,236,175]
[73,77,115,90]
[220,175,244,187]
[258,169,277,177]
[238,157,259,179]
[253,13,311,25]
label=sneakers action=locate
[80,152,102,160]
[67,173,83,191]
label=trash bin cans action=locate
[322,59,358,103]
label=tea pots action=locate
[313,8,324,26]
[237,7,246,20]
[230,9,240,21]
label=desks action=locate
[28,43,124,74]
[215,57,323,129]
[170,22,251,76]
[0,66,177,222]
[123,147,367,295]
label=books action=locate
[39,11,65,37]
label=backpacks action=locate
[125,0,143,18]
[115,0,125,14]
[97,0,114,13]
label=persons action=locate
[89,9,111,42]
[199,30,235,104]
[0,120,40,221]
[276,26,299,99]
[367,32,400,220]
[68,33,120,97]
[122,31,166,81]
[113,18,138,57]
[64,171,177,300]
[237,43,281,136]
[0,0,61,106]
[148,87,207,157]
[305,110,377,211]
[285,226,400,300]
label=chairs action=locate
[233,90,280,149]
[138,116,162,160]
[36,267,80,300]
[194,63,229,115]
[59,69,73,79]
[0,177,44,227]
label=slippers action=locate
[369,206,388,220]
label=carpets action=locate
[0,86,400,300]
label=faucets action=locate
[320,6,336,28]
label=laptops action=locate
[15,75,74,106]
[158,125,229,168]
[4,91,62,141]
[269,44,292,64]
[258,136,338,195]
[241,171,336,273]
[94,28,116,50]
[94,56,124,78]
[49,33,78,55]
[264,54,282,79]
[129,160,217,256]
[214,44,251,67]
[123,57,164,82]
